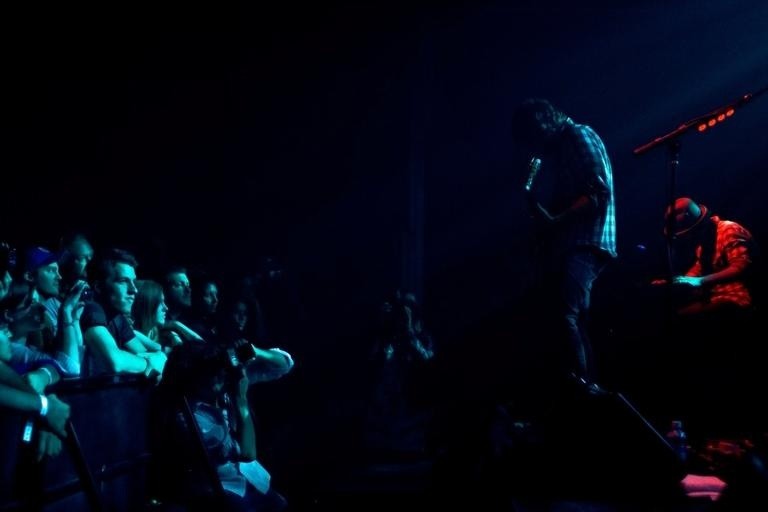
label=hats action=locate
[664,197,708,237]
[24,246,69,272]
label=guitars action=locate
[523,156,562,256]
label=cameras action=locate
[222,340,258,372]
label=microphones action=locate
[523,158,542,195]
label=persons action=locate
[358,289,434,473]
[513,97,618,372]
[649,197,753,314]
[0,234,295,511]
